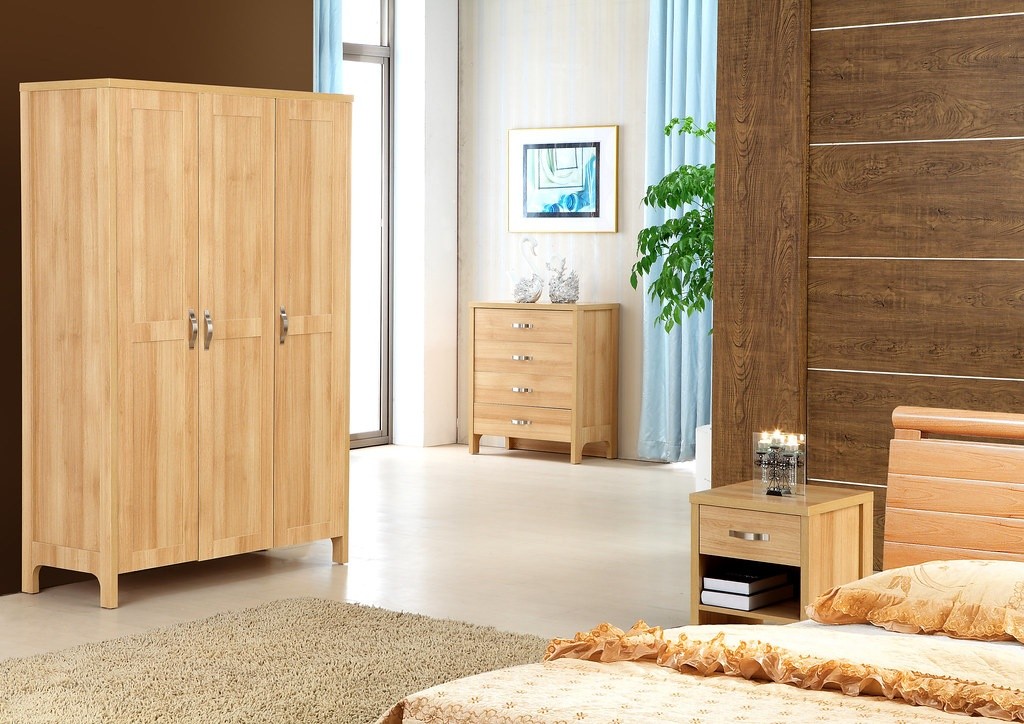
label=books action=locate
[701,583,796,614]
[701,562,790,597]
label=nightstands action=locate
[689,479,874,629]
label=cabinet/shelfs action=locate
[20,77,355,608]
[469,300,620,465]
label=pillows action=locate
[807,560,1023,644]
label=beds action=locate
[375,405,1024,724]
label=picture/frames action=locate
[508,125,619,233]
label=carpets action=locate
[0,598,561,724]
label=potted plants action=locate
[630,116,715,492]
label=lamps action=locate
[751,430,807,498]
